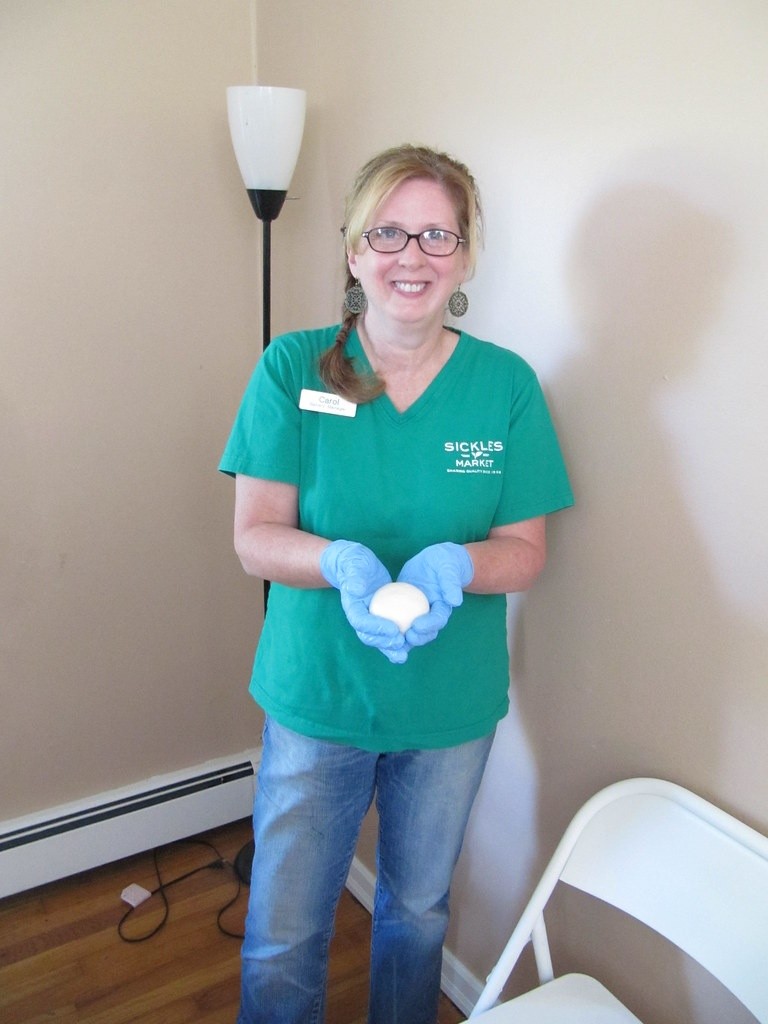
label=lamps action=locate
[225,86,308,885]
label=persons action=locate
[234,143,547,1024]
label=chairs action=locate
[451,778,767,1024]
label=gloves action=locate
[395,541,474,648]
[319,538,408,665]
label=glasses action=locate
[353,227,469,258]
